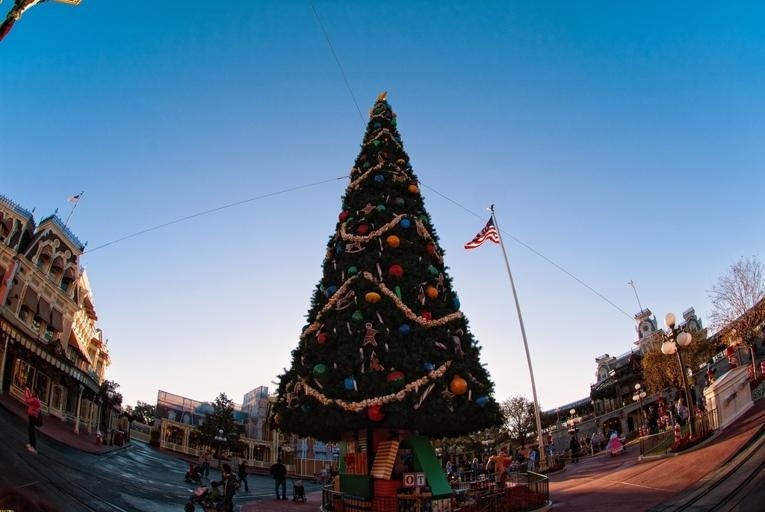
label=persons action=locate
[319,466,339,485]
[24,386,41,455]
[446,431,627,482]
[643,398,687,435]
[184,448,251,512]
[97,430,104,444]
[270,459,288,500]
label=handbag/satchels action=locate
[35,410,44,428]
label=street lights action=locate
[660,311,700,442]
[632,382,649,457]
[567,408,583,442]
[555,407,562,429]
[728,326,744,367]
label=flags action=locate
[464,216,500,249]
[68,192,84,203]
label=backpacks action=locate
[226,472,242,491]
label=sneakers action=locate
[25,443,38,456]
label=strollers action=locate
[184,462,204,485]
[184,480,224,512]
[291,476,308,504]
